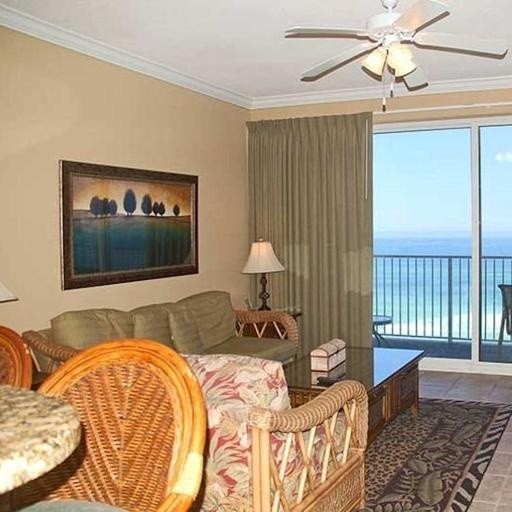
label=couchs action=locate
[22,292,300,381]
[175,354,366,512]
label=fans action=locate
[283,0,509,90]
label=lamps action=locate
[362,35,419,80]
[240,236,285,311]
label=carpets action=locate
[359,397,512,512]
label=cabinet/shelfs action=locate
[237,307,302,346]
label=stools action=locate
[372,314,394,348]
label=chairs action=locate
[0,326,32,390]
[4,339,207,512]
[495,282,512,362]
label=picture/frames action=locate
[58,159,199,292]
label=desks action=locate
[0,384,80,497]
